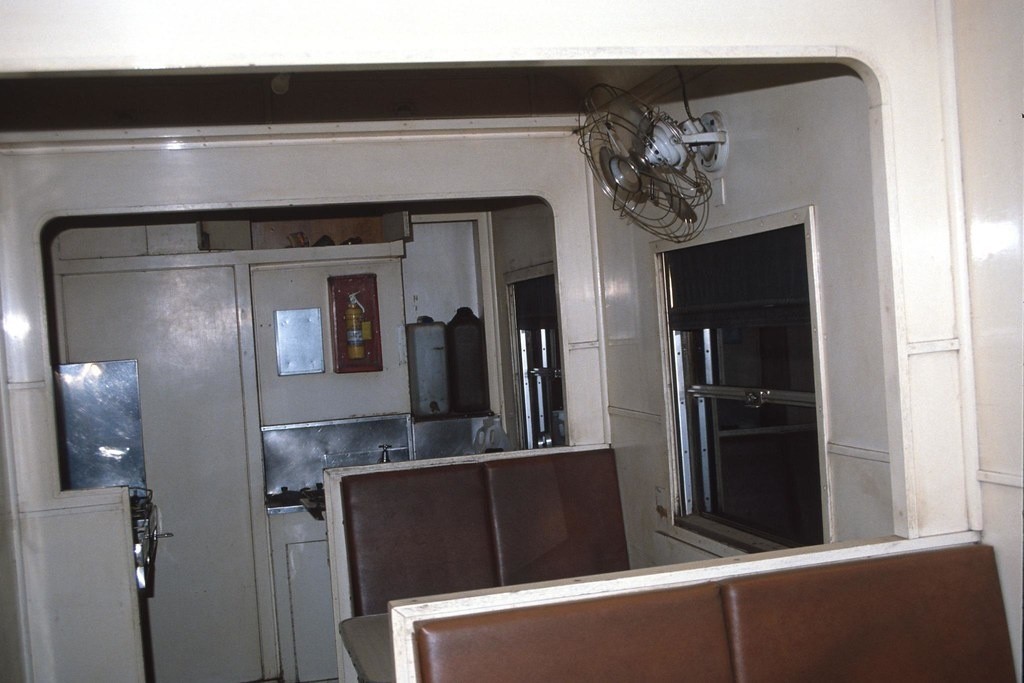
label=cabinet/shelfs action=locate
[44,199,500,683]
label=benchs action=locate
[389,527,1018,683]
[321,444,630,682]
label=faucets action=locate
[376,443,393,464]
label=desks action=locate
[338,614,394,683]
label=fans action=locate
[574,82,731,244]
